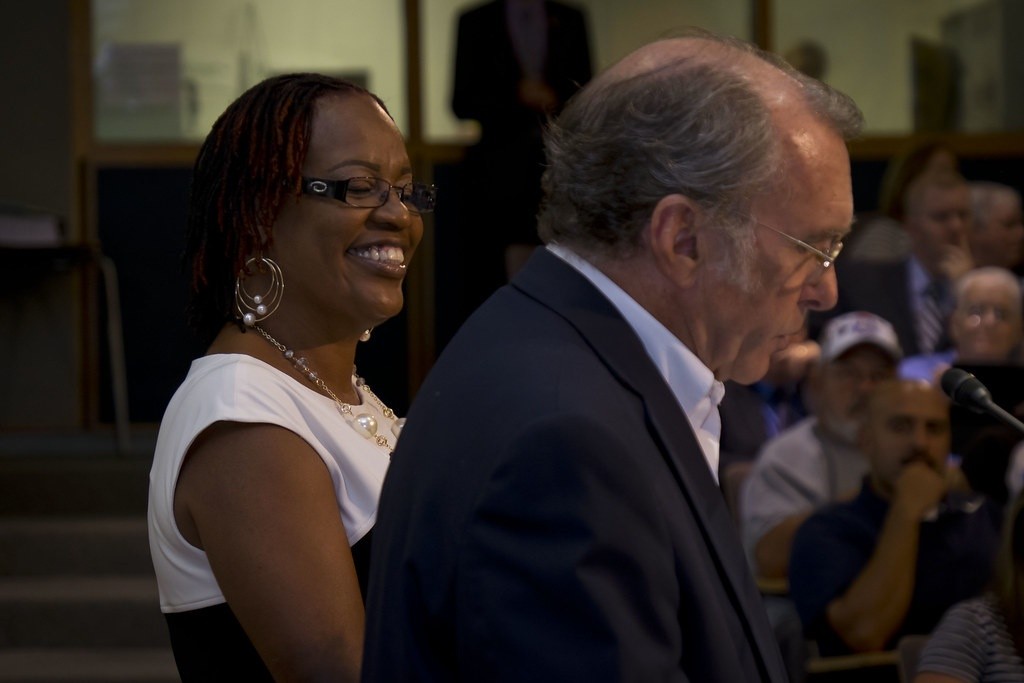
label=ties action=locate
[925,281,954,355]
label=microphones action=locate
[941,368,1024,433]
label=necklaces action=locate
[237,311,407,453]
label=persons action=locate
[452,0,592,286]
[725,135,1024,683]
[147,71,440,683]
[784,39,825,75]
[901,263,1024,382]
[741,309,906,579]
[361,24,867,683]
[791,375,967,682]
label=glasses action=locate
[711,202,842,285]
[281,176,438,213]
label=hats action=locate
[820,312,903,364]
